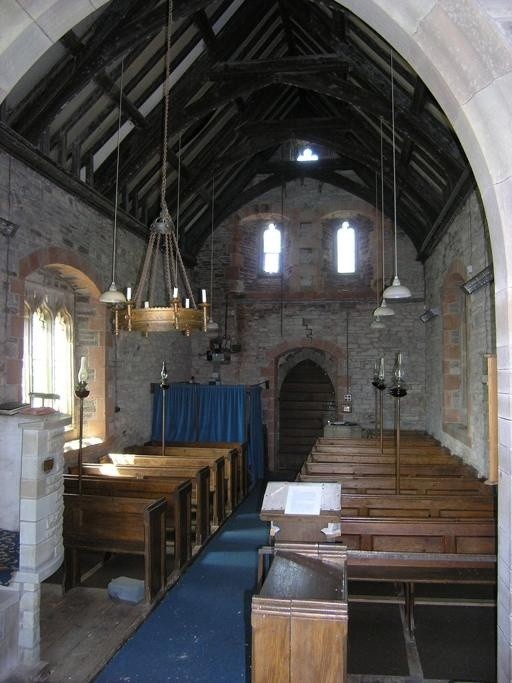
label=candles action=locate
[144,301,150,308]
[202,289,207,302]
[126,287,131,300]
[173,288,178,299]
[185,299,190,308]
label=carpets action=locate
[91,469,300,682]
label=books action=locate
[1,402,32,417]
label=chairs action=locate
[257,517,498,631]
[68,462,211,545]
[369,429,426,435]
[124,445,239,509]
[368,434,433,440]
[300,461,478,478]
[62,492,168,604]
[144,440,248,497]
[341,493,499,522]
[295,472,478,494]
[311,445,450,455]
[62,473,193,570]
[315,437,441,444]
[307,451,462,465]
[98,453,226,526]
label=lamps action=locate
[388,352,409,495]
[383,49,412,299]
[99,56,127,303]
[370,171,387,329]
[160,360,170,456]
[110,0,212,339]
[75,356,90,494]
[372,361,379,439]
[377,357,388,458]
[206,175,219,328]
[374,114,396,316]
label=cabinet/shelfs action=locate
[0,413,72,584]
[249,542,349,683]
